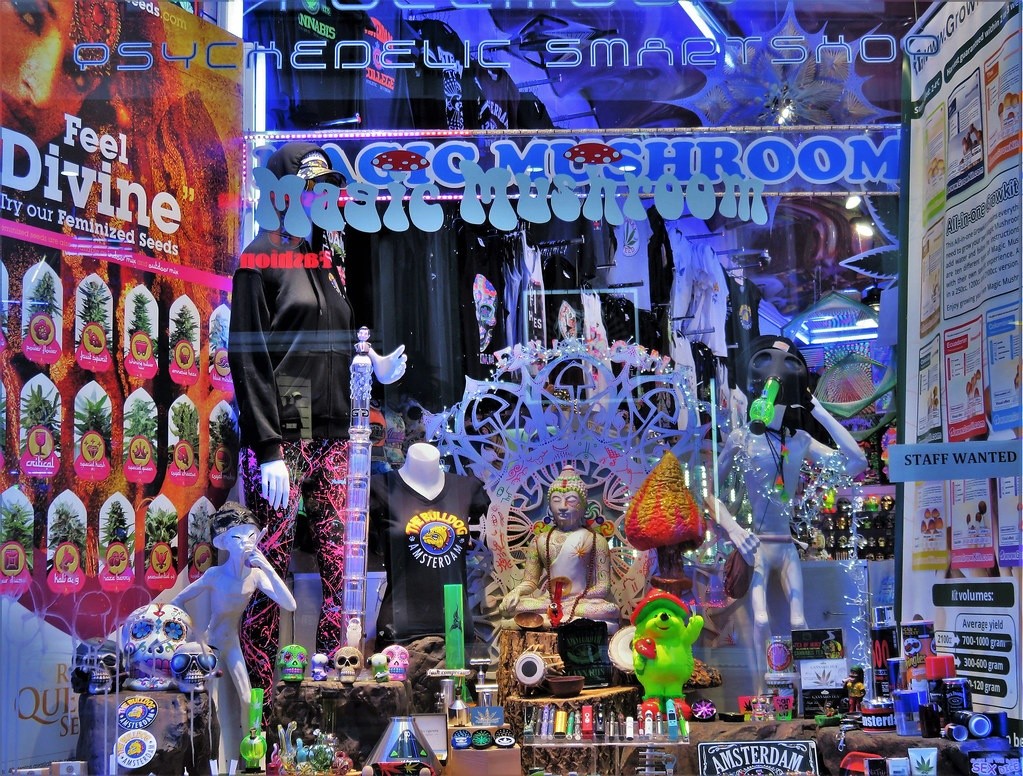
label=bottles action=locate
[887,658,992,742]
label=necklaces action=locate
[541,525,596,624]
[765,425,786,488]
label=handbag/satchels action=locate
[722,549,755,600]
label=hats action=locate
[734,334,814,423]
[296,151,348,189]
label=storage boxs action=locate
[448,723,521,776]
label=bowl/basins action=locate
[514,611,543,630]
[546,676,585,698]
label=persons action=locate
[367,442,488,647]
[173,501,297,775]
[0,0,125,163]
[704,336,869,695]
[498,465,620,632]
[227,141,407,774]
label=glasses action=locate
[169,652,218,676]
[303,178,337,192]
